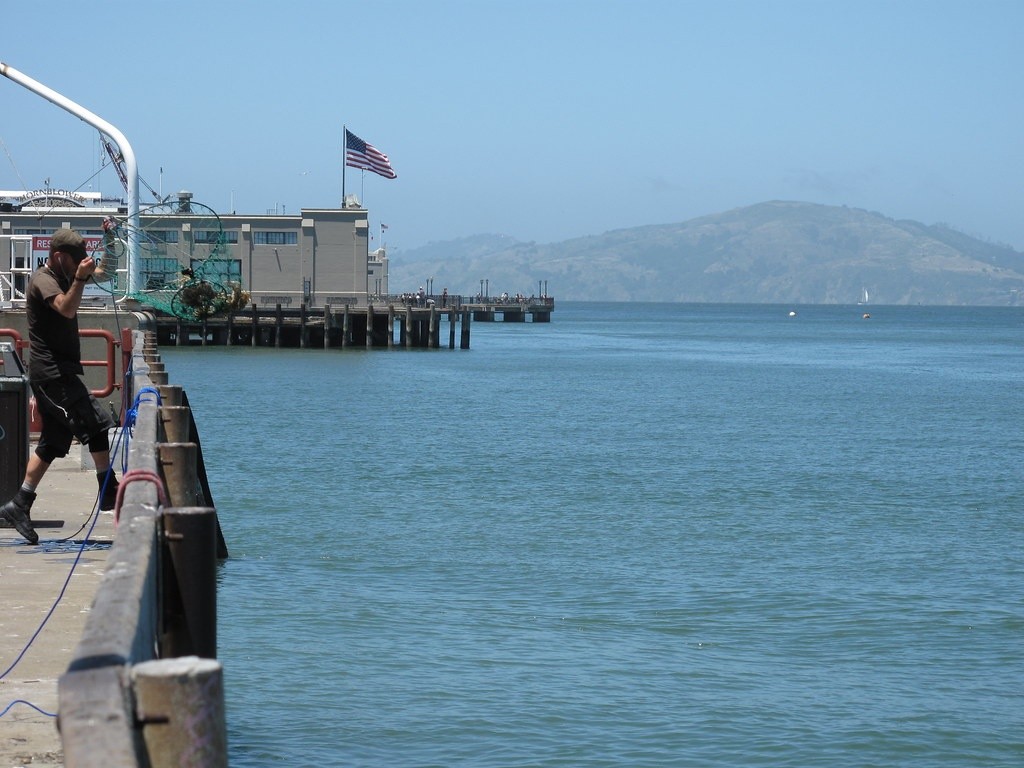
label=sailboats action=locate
[858,286,871,305]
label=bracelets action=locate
[74,276,88,282]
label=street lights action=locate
[426,278,433,296]
[539,280,548,306]
[480,279,489,297]
[375,279,382,300]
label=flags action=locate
[381,223,389,233]
[369,232,373,241]
[346,128,397,179]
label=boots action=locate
[97,468,125,511]
[0,488,38,542]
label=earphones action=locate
[58,257,61,264]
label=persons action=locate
[0,217,126,544]
[442,287,449,308]
[477,293,547,306]
[401,286,426,308]
